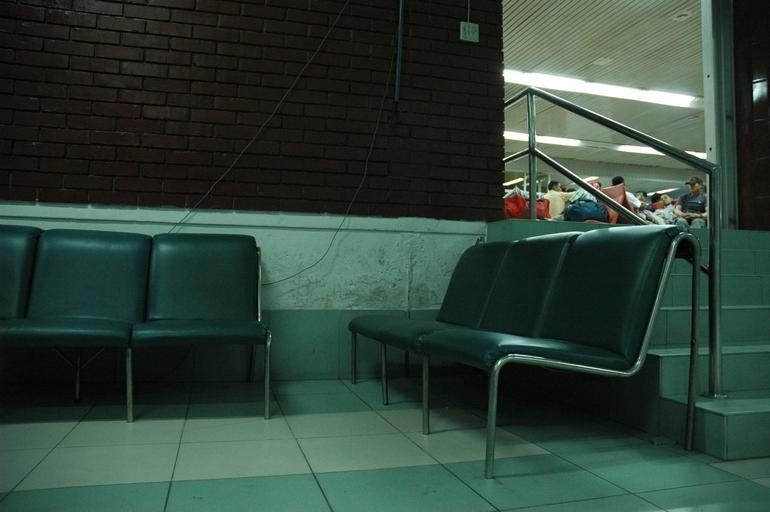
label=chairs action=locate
[378,231,582,413]
[0,224,153,421]
[349,239,517,385]
[126,232,271,421]
[419,226,704,480]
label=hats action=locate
[685,176,704,185]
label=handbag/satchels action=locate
[503,195,552,220]
[564,198,611,223]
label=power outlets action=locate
[461,21,479,43]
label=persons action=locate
[541,176,707,229]
